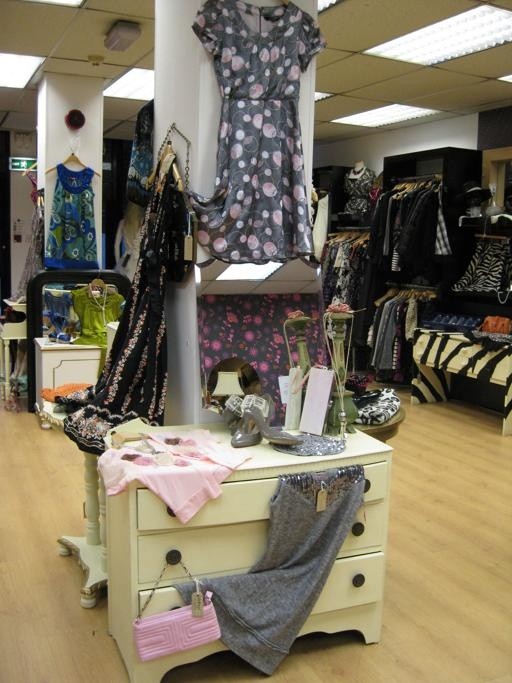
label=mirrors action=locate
[207,357,262,416]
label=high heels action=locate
[231,406,298,448]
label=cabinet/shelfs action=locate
[101,422,393,682]
[34,338,106,430]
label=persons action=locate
[343,160,376,222]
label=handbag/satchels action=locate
[477,316,511,334]
[132,561,221,663]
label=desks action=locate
[410,327,512,436]
[1,297,26,402]
[353,405,406,444]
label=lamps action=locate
[210,371,245,404]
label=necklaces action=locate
[352,166,366,175]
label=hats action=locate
[451,179,492,207]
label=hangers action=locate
[69,267,115,297]
[20,148,99,220]
[325,224,373,248]
[388,174,442,201]
[150,143,188,193]
[372,284,433,306]
